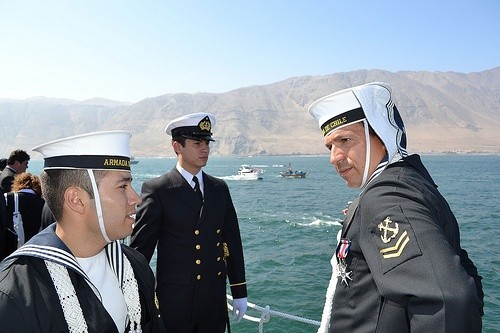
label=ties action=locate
[192,176,202,201]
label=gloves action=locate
[232,297,247,322]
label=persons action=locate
[0,149,55,262]
[128,113,247,333]
[309,82,484,333]
[0,130,167,333]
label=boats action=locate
[236,166,263,180]
[280,163,306,179]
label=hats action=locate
[31,130,132,243]
[308,82,408,188]
[165,113,216,142]
